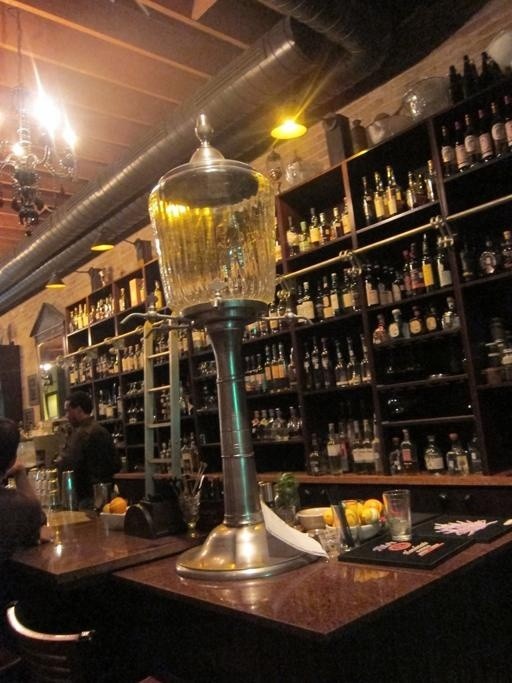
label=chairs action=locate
[4,598,103,682]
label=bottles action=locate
[282,154,437,256]
[246,228,511,472]
[437,45,512,173]
[67,270,216,471]
[258,472,300,523]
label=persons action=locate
[54,390,122,502]
[0,415,50,561]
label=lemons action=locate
[323,499,383,528]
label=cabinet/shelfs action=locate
[62,75,511,531]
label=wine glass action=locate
[175,475,204,540]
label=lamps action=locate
[44,265,108,296]
[0,0,81,241]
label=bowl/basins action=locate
[98,509,129,530]
[295,505,336,530]
[323,522,379,541]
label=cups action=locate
[93,482,113,512]
[33,464,60,508]
[61,469,80,509]
[381,486,412,543]
